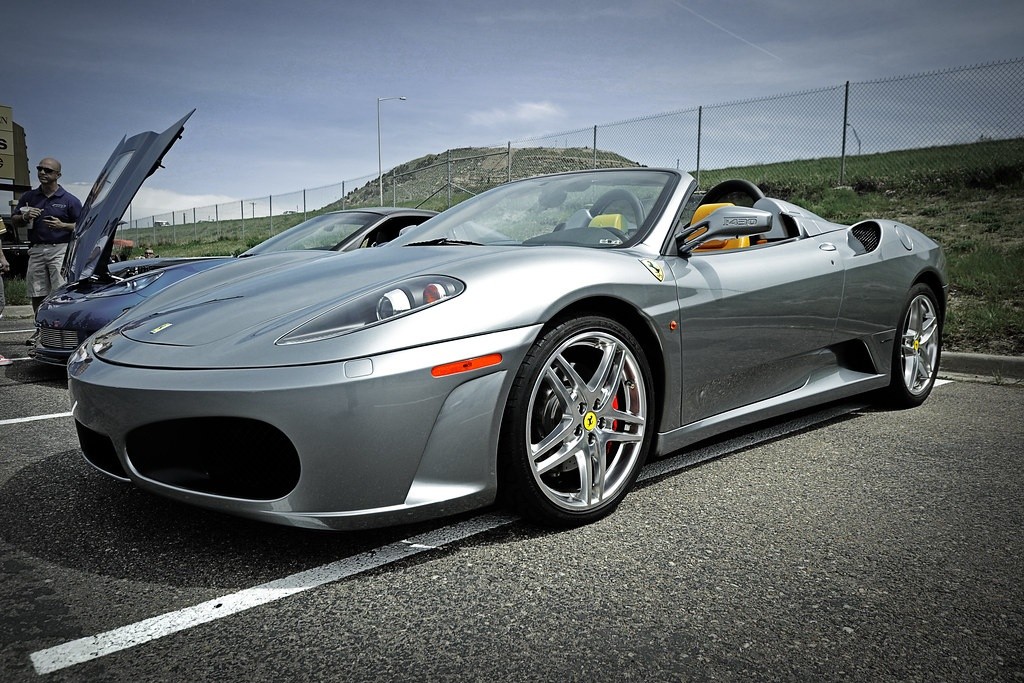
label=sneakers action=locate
[0,354,13,365]
[26,330,39,346]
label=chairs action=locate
[684,203,750,252]
[587,214,628,234]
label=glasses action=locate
[145,252,153,255]
[36,166,60,174]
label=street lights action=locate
[377,95,409,206]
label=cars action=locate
[154,221,171,227]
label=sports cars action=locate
[26,110,511,369]
[69,166,949,530]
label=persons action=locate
[79,217,118,280]
[139,248,153,259]
[11,158,82,346]
[0,217,13,365]
